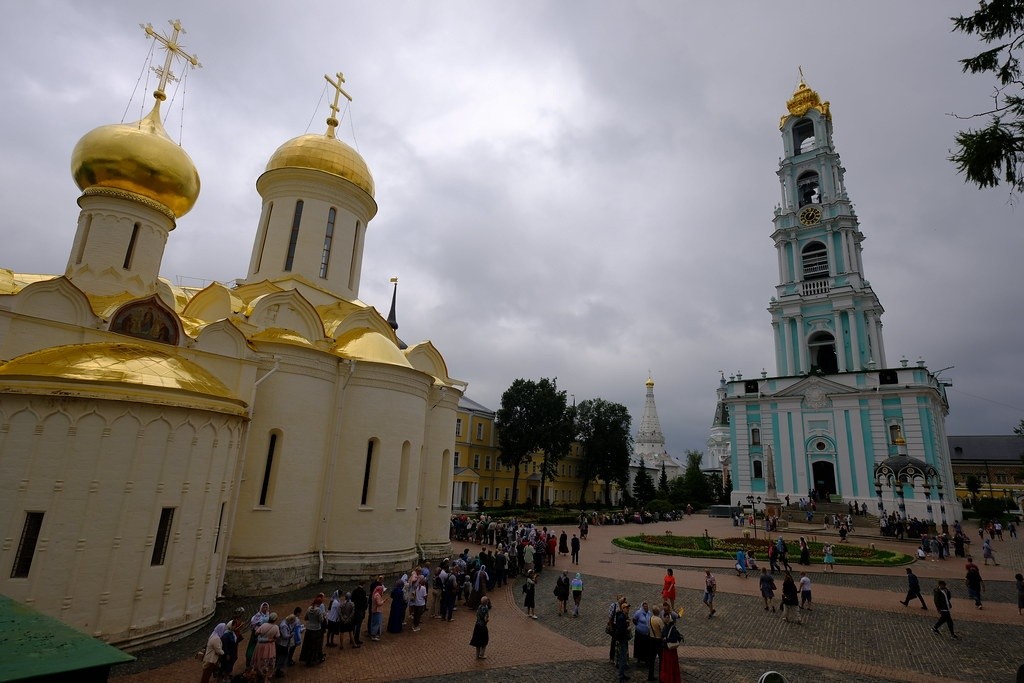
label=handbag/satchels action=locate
[605,602,617,635]
[667,625,680,650]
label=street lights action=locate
[747,495,762,539]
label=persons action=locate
[199,487,1024,683]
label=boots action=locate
[572,605,581,616]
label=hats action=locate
[621,602,632,609]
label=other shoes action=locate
[783,617,788,622]
[900,600,909,606]
[950,635,962,641]
[931,627,942,636]
[448,618,455,622]
[412,626,421,632]
[799,605,804,609]
[736,574,741,577]
[441,618,447,621]
[476,655,487,660]
[922,605,927,610]
[764,606,769,611]
[430,614,442,619]
[797,619,802,625]
[528,614,538,619]
[707,609,717,620]
[745,573,748,579]
[807,608,813,611]
[975,604,983,609]
[275,671,285,678]
[772,606,776,612]
[287,661,297,667]
[326,643,338,648]
[372,635,385,640]
